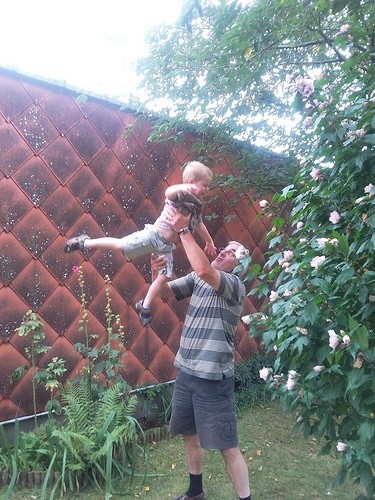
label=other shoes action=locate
[135,299,153,328]
[175,491,205,500]
[63,233,90,253]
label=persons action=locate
[150,206,252,500]
[64,161,214,327]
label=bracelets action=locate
[178,227,189,235]
